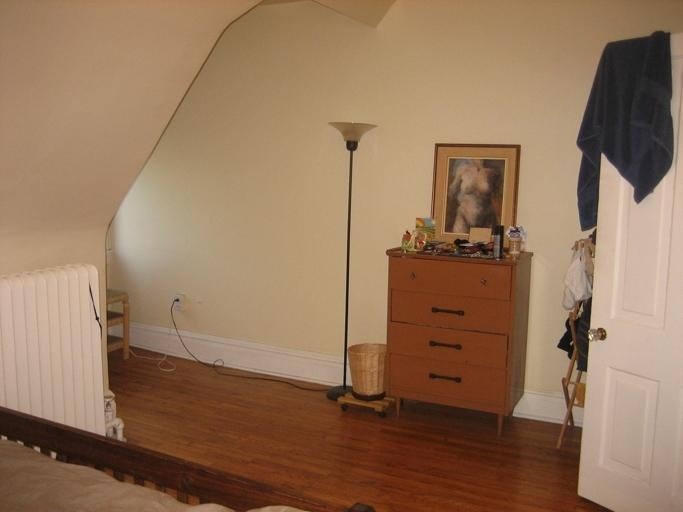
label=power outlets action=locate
[174,295,183,309]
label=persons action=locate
[447,158,502,234]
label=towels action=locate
[576,31,674,232]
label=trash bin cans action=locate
[347,343,386,401]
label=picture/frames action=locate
[431,143,521,246]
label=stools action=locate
[106,290,130,360]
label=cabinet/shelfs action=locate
[384,247,534,438]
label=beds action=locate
[0,405,376,512]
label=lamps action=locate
[327,122,378,406]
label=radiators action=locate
[0,263,106,440]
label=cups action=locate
[509,237,522,256]
